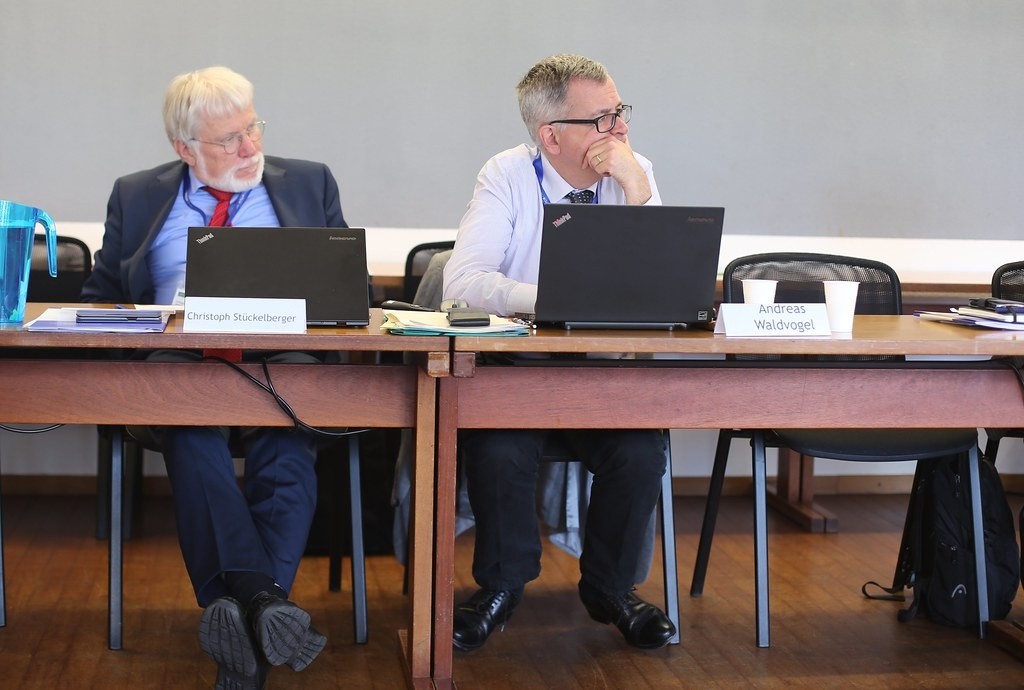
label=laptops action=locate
[514,203,725,331]
[184,226,369,327]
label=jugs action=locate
[0,200,57,325]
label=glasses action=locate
[190,120,265,154]
[549,104,632,133]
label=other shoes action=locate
[248,592,327,672]
[197,593,273,690]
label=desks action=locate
[433,315,1024,690]
[367,277,826,535]
[0,303,449,690]
[802,286,991,534]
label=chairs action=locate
[0,234,1024,647]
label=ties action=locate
[550,190,595,360]
[199,186,242,363]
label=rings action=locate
[596,155,601,162]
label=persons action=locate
[442,54,678,651]
[75,67,370,689]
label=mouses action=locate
[440,299,469,313]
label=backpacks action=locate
[862,444,1021,629]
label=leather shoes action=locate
[452,585,524,652]
[578,574,677,649]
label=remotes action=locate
[381,299,436,312]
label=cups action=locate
[742,279,780,303]
[822,280,861,334]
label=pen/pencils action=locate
[513,317,537,330]
[116,304,127,308]
